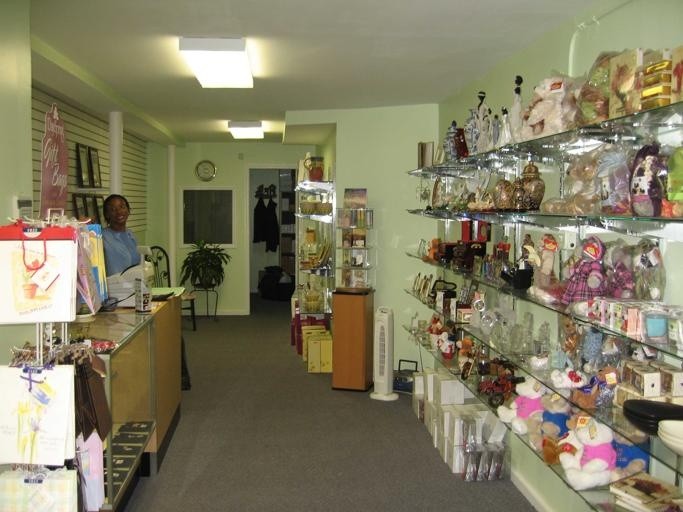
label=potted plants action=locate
[179,239,232,290]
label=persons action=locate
[79,193,143,278]
[522,234,534,259]
[478,76,523,152]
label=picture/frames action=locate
[72,143,109,228]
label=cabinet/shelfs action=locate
[288,154,375,393]
[399,103,683,511]
[53,286,184,512]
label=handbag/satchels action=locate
[0,223,108,324]
[0,339,113,512]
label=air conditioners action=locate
[370,306,400,401]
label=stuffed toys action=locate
[518,55,611,140]
[429,314,472,363]
[564,237,664,332]
[529,234,555,295]
[542,136,683,218]
[497,374,648,490]
[532,318,653,410]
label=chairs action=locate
[145,246,196,331]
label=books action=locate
[610,472,674,512]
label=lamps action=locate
[179,33,264,140]
